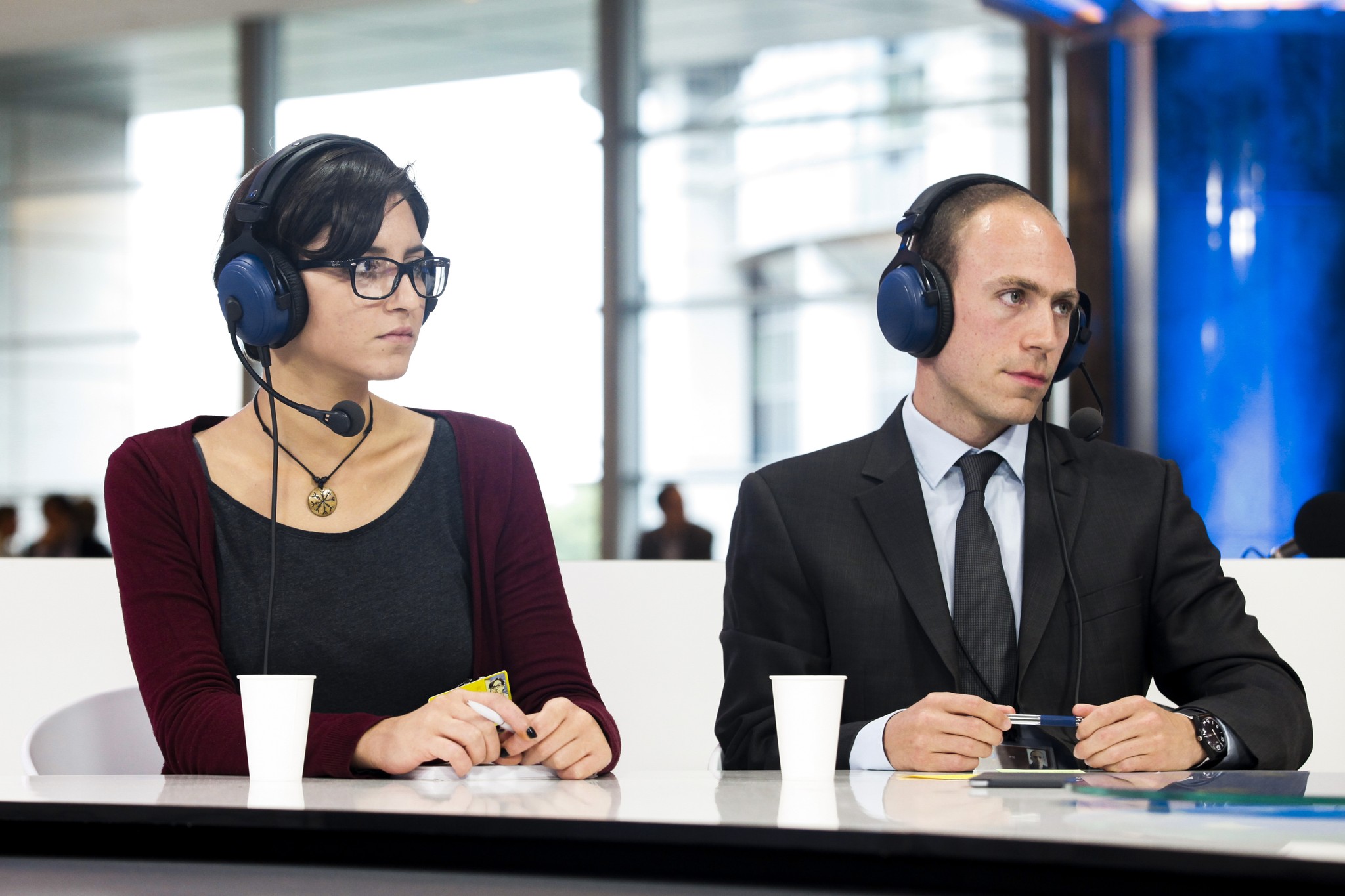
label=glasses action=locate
[296,256,450,301]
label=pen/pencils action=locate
[463,697,518,733]
[966,712,1087,728]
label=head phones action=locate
[877,174,1092,387]
[212,133,437,351]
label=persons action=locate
[1029,750,1048,769]
[103,131,623,780]
[713,173,1315,773]
[635,485,713,559]
[488,677,509,699]
[0,492,111,557]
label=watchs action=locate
[1175,707,1228,771]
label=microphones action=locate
[1068,357,1105,440]
[222,296,367,438]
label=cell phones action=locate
[968,771,1069,789]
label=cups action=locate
[768,673,847,785]
[235,671,317,784]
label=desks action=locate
[0,769,1345,891]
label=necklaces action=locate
[253,388,373,517]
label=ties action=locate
[951,451,1023,742]
